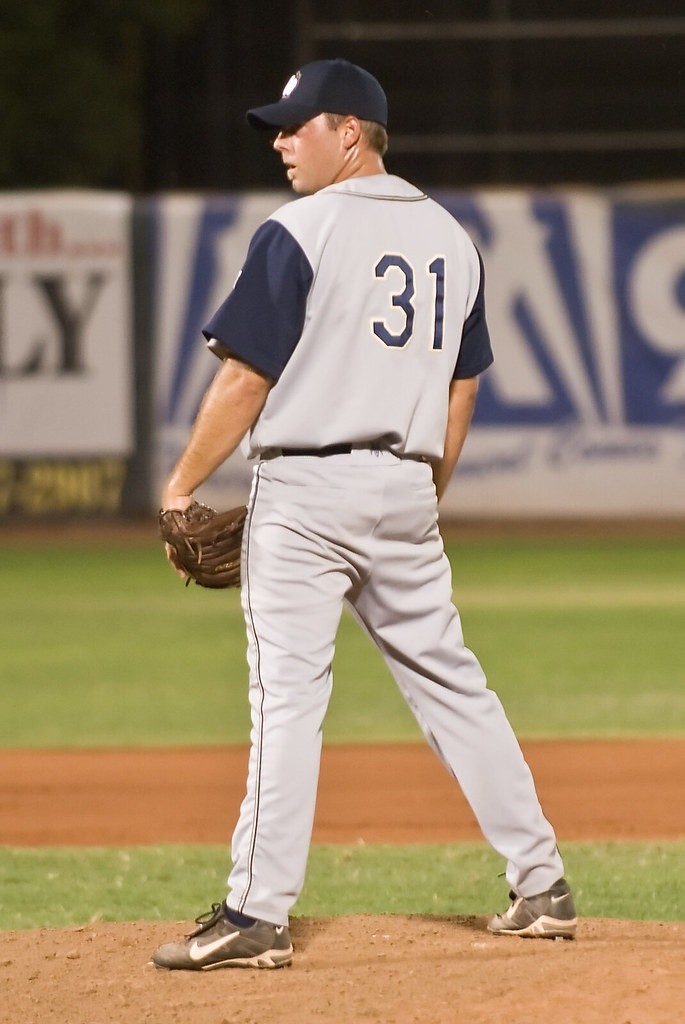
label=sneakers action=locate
[150,899,293,969]
[487,872,577,939]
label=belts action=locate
[282,442,351,457]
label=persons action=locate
[148,60,578,971]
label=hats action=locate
[245,60,388,129]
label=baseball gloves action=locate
[152,498,254,589]
